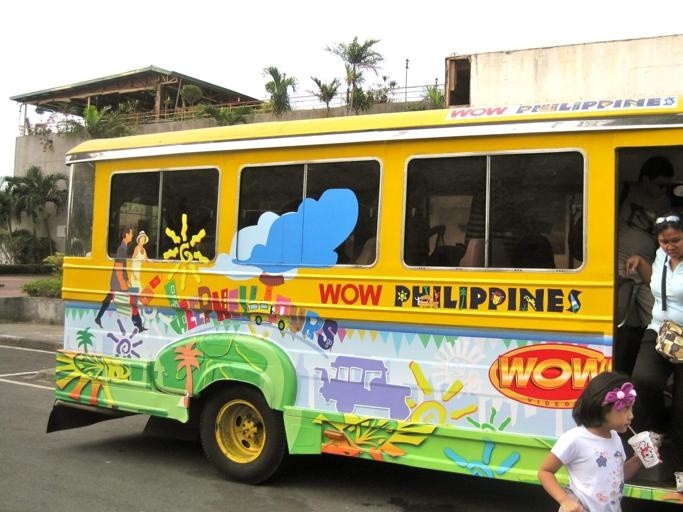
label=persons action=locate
[538,372,663,512]
[464,152,683,474]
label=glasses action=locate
[656,215,680,224]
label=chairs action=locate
[356,237,376,265]
[458,238,486,266]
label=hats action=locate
[136,231,149,244]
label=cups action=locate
[628,431,660,470]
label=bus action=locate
[46,104,679,506]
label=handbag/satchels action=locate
[655,320,683,364]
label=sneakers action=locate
[675,471,683,491]
